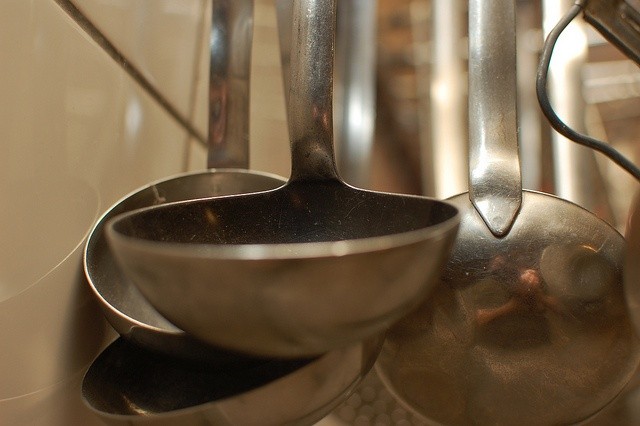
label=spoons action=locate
[82,1,291,336]
[106,0,461,355]
[66,323,382,424]
[369,0,635,426]
